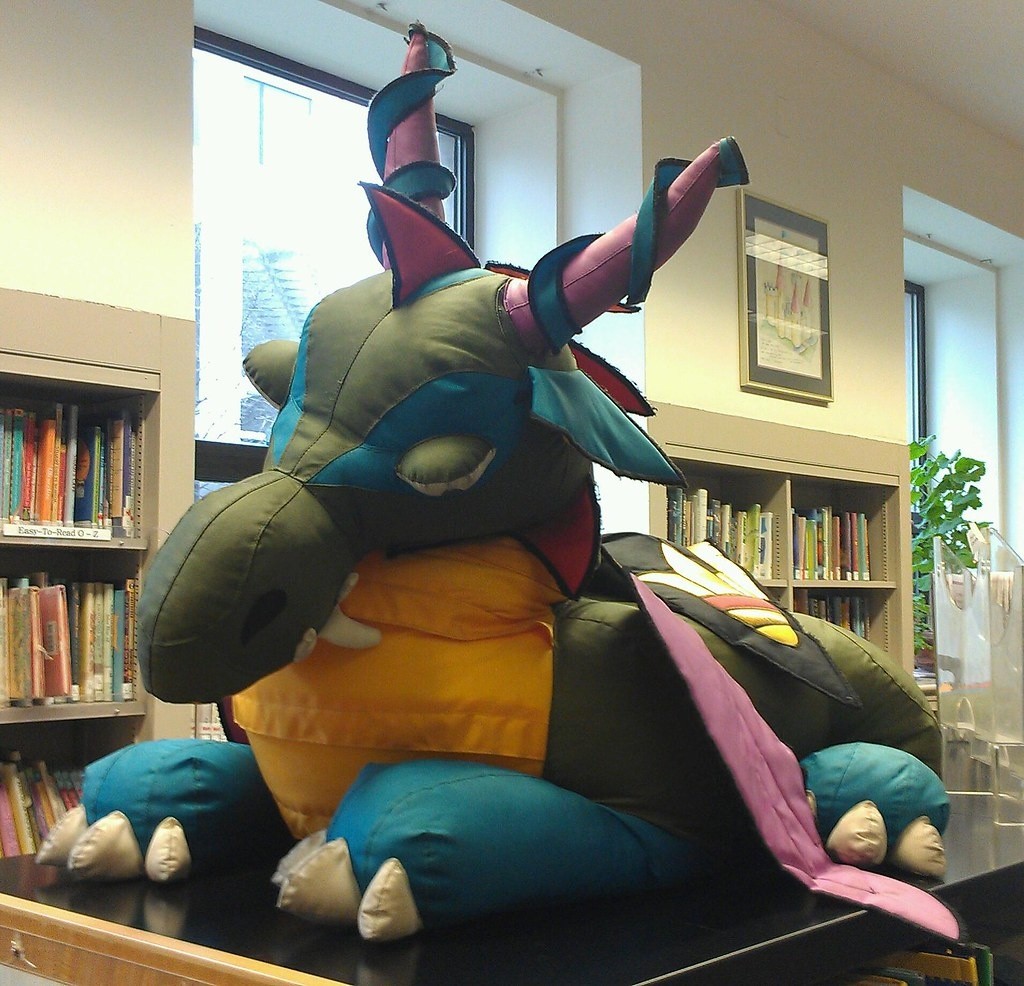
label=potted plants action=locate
[895,429,993,678]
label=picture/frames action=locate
[735,186,835,406]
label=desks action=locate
[1,741,1024,986]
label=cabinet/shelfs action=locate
[0,285,197,868]
[645,398,914,684]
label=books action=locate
[793,588,871,642]
[196,702,229,743]
[792,505,871,581]
[0,751,86,887]
[666,483,773,580]
[0,571,134,707]
[0,402,136,542]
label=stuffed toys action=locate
[35,15,961,943]
